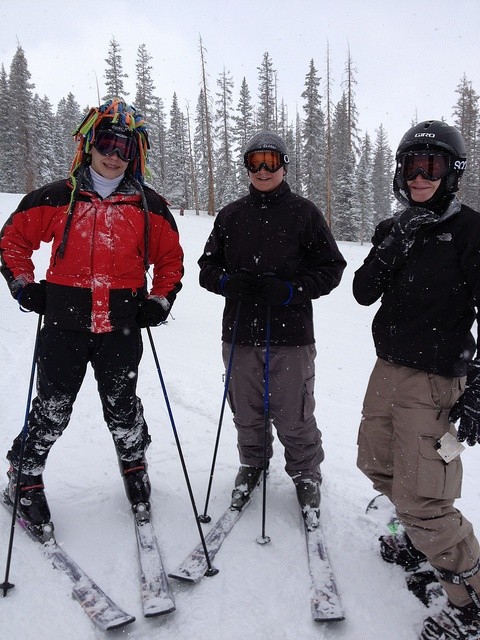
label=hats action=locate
[243,132,289,172]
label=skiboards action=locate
[0,484,176,631]
[168,505,346,622]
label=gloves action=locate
[255,275,293,307]
[136,295,169,328]
[375,206,441,271]
[214,270,253,298]
[448,378,480,446]
[18,282,48,317]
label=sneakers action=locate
[121,467,151,503]
[420,600,479,640]
[234,465,261,494]
[296,474,322,508]
[379,532,425,565]
[15,474,51,525]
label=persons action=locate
[1,96,183,542]
[197,132,347,533]
[353,120,480,640]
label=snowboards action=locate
[366,492,480,640]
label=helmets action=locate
[391,119,467,212]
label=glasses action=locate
[396,150,452,182]
[91,130,138,161]
[244,151,281,173]
